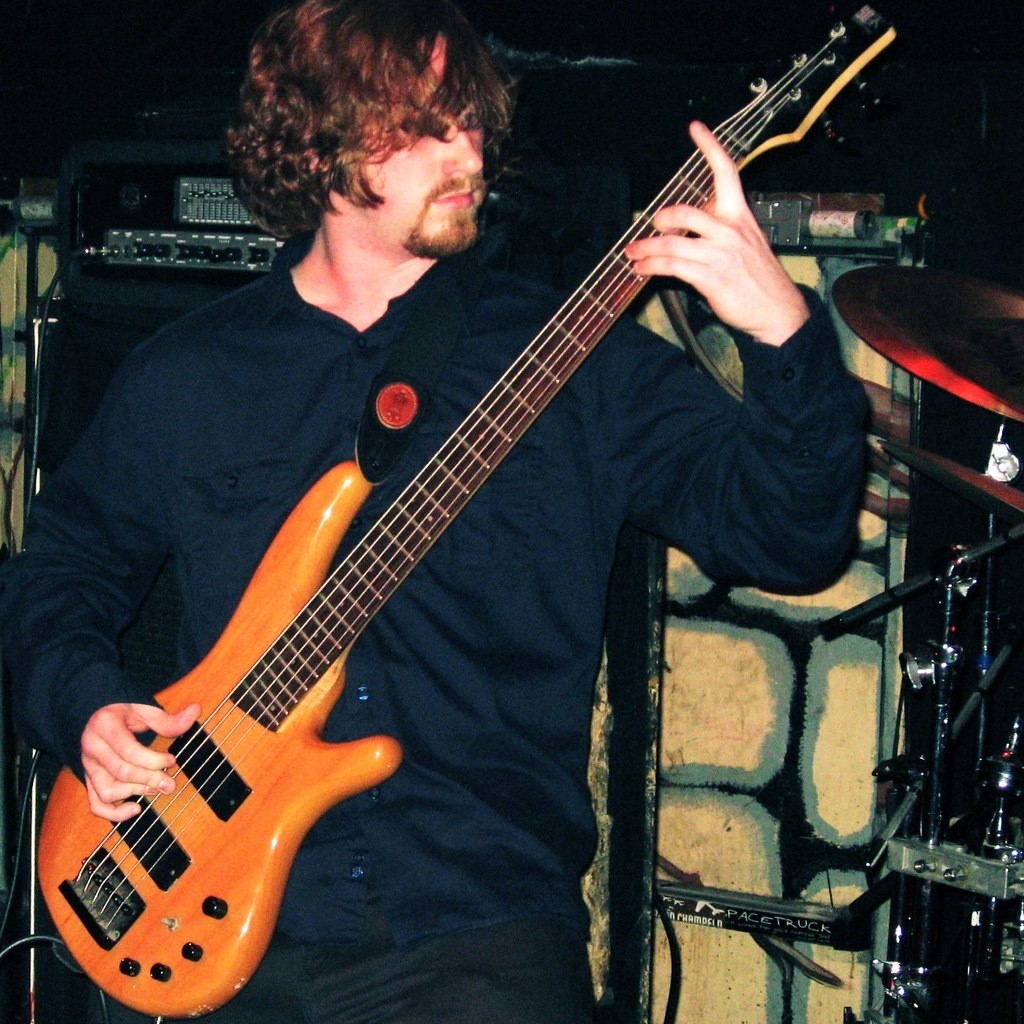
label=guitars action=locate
[35,1,901,1023]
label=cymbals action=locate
[831,263,1024,423]
[873,438,1024,520]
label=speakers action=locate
[23,310,189,520]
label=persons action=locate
[1,1,876,1023]
